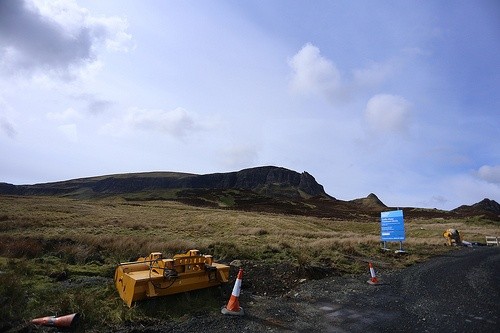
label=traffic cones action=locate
[367,261,384,286]
[222,268,247,316]
[32,312,81,331]
